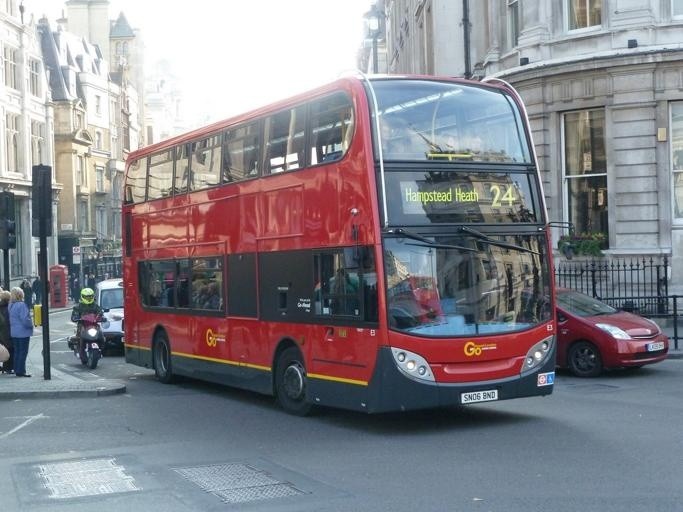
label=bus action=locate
[118,70,574,419]
[385,274,444,327]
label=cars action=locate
[453,284,668,378]
[93,277,127,355]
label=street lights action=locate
[426,248,434,277]
[87,248,102,280]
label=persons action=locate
[7,286,34,377]
[20,271,95,309]
[0,290,12,374]
[70,287,104,351]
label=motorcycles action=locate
[65,303,109,369]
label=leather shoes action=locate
[2,368,31,377]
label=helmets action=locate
[81,288,94,305]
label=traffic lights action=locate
[0,218,18,252]
